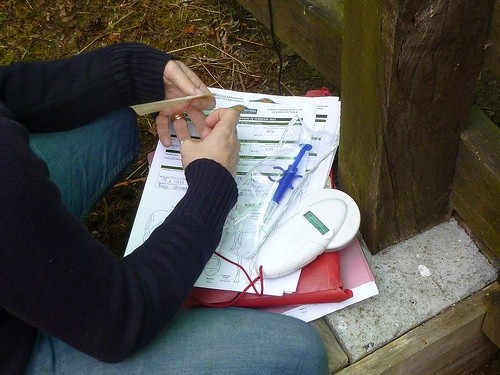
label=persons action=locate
[1,41,329,375]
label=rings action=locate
[171,112,188,123]
[181,140,185,146]
